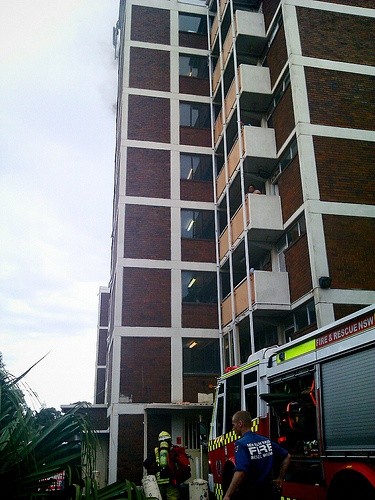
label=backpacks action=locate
[166,444,192,487]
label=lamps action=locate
[186,219,195,232]
[187,277,197,289]
[186,167,193,180]
[188,341,198,349]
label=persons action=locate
[223,411,291,500]
[248,184,262,194]
[143,431,191,500]
[236,119,251,129]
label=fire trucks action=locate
[199,304,375,500]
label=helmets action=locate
[158,431,172,442]
